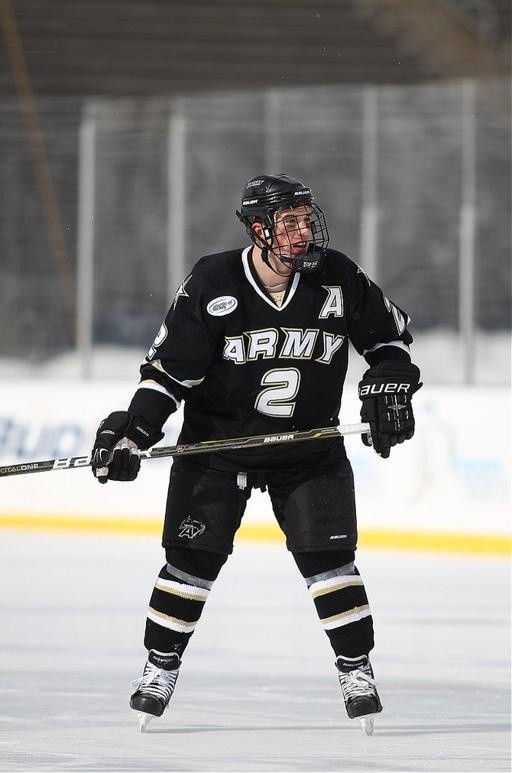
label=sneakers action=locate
[130,650,182,717]
[335,655,382,718]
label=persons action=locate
[92,175,419,719]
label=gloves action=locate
[91,411,164,484]
[359,361,422,458]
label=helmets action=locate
[236,173,329,272]
